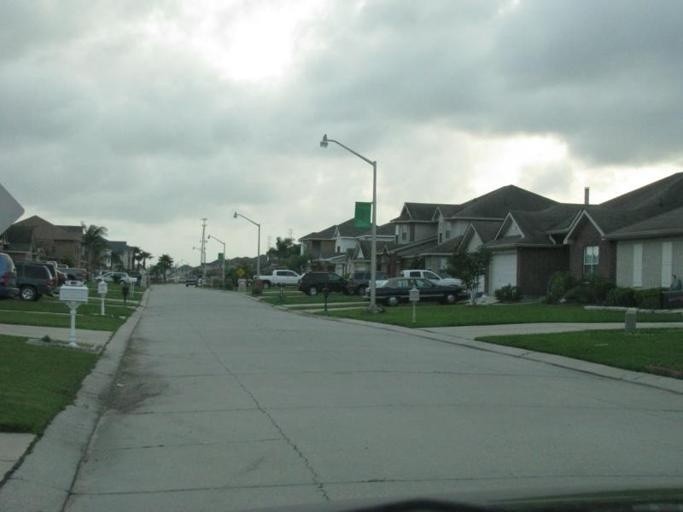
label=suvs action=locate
[293,268,468,306]
[0,250,139,303]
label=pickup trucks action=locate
[252,269,300,289]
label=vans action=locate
[185,274,198,287]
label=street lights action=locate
[318,132,378,310]
[232,210,261,283]
[191,246,208,279]
[207,234,226,288]
